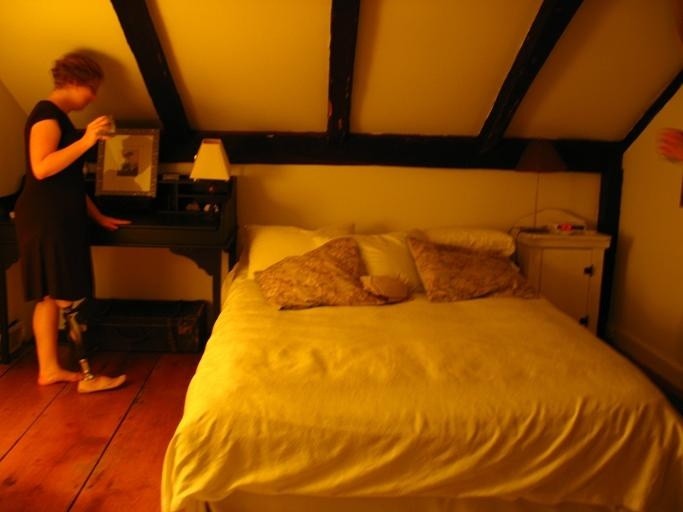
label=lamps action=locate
[189,138,232,213]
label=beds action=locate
[160,240,683,512]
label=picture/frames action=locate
[94,126,160,198]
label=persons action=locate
[14,48,135,392]
[118,148,137,177]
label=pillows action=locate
[243,225,515,310]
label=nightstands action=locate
[515,226,614,336]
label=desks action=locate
[86,171,238,351]
